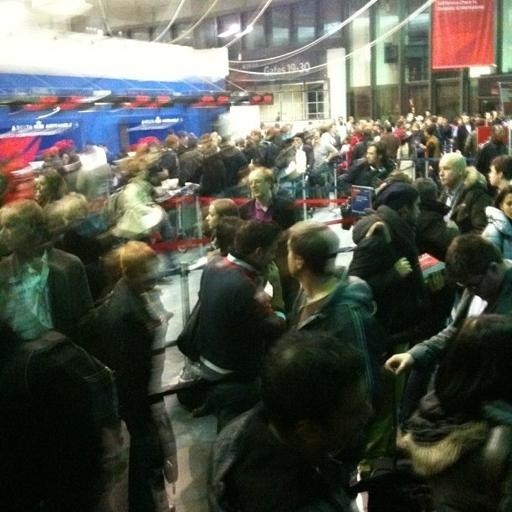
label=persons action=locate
[0,106,512,511]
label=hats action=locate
[372,181,416,212]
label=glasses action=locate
[455,275,485,292]
